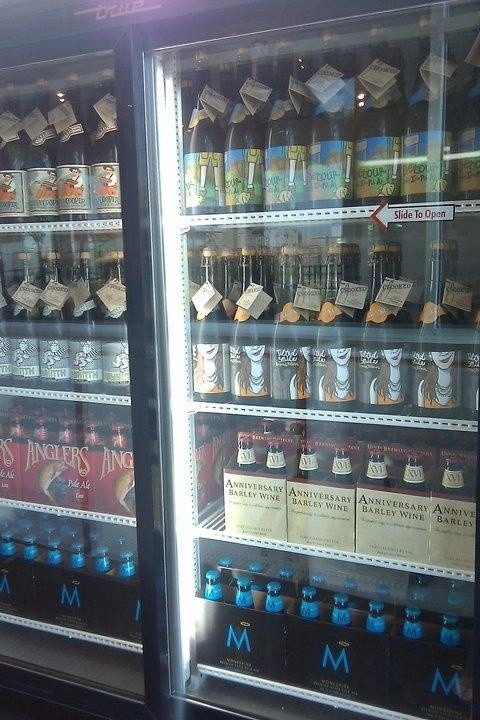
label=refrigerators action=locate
[2,5,476,717]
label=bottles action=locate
[2,29,474,713]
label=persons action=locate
[1,166,120,202]
[193,342,471,414]
[198,134,451,191]
